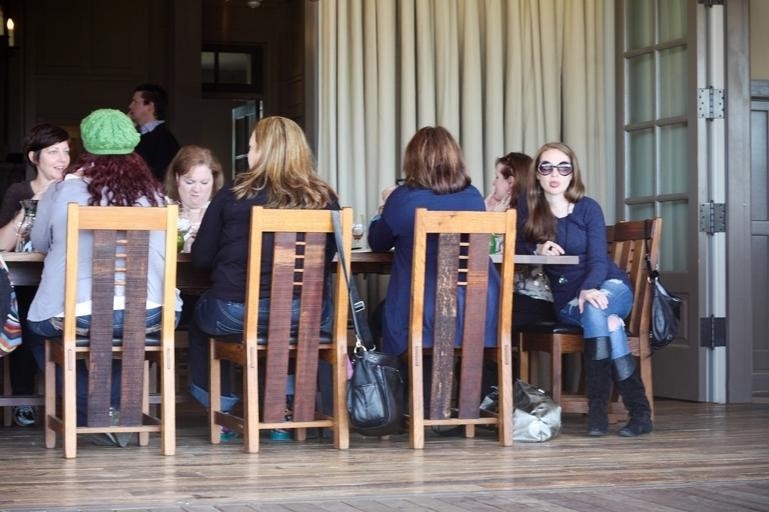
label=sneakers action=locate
[14,406,36,427]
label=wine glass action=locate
[352,224,364,249]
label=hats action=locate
[81,109,142,156]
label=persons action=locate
[484,140,653,435]
[123,86,179,176]
[187,115,342,445]
[369,125,499,431]
[164,145,224,244]
[1,109,184,427]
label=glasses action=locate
[538,161,573,176]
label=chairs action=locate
[372,203,523,450]
[206,204,360,456]
[513,213,670,425]
[40,200,181,459]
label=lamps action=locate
[4,18,16,49]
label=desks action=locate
[0,247,583,430]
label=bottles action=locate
[16,200,39,253]
[178,224,195,254]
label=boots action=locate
[611,353,652,436]
[583,336,610,436]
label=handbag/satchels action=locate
[479,380,562,442]
[351,351,408,436]
[647,270,682,347]
